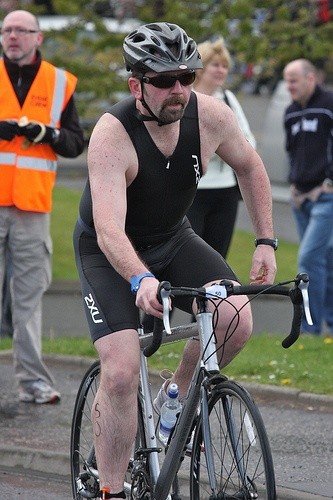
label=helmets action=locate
[122,22,203,73]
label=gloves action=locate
[0,120,55,145]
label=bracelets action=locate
[127,272,155,295]
[254,237,279,251]
[325,178,332,194]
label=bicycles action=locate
[68,271,314,500]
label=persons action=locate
[282,58,333,338]
[73,21,278,500]
[0,10,86,404]
[186,38,257,260]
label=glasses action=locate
[136,72,195,88]
[0,26,37,36]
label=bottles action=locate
[159,384,182,444]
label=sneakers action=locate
[153,369,204,452]
[19,379,61,403]
